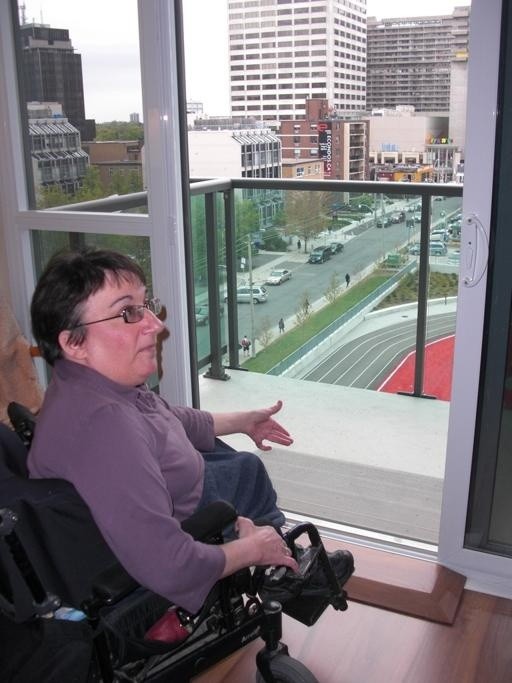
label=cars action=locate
[374,194,462,258]
[195,301,225,326]
[266,268,293,286]
[329,242,345,255]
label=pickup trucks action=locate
[332,201,350,211]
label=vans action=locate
[223,285,269,305]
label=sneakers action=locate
[260,545,353,601]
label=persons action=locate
[278,317,286,334]
[22,240,356,617]
[240,334,251,357]
[344,273,351,287]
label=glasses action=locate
[68,297,162,330]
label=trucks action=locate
[309,246,331,264]
[351,204,373,216]
